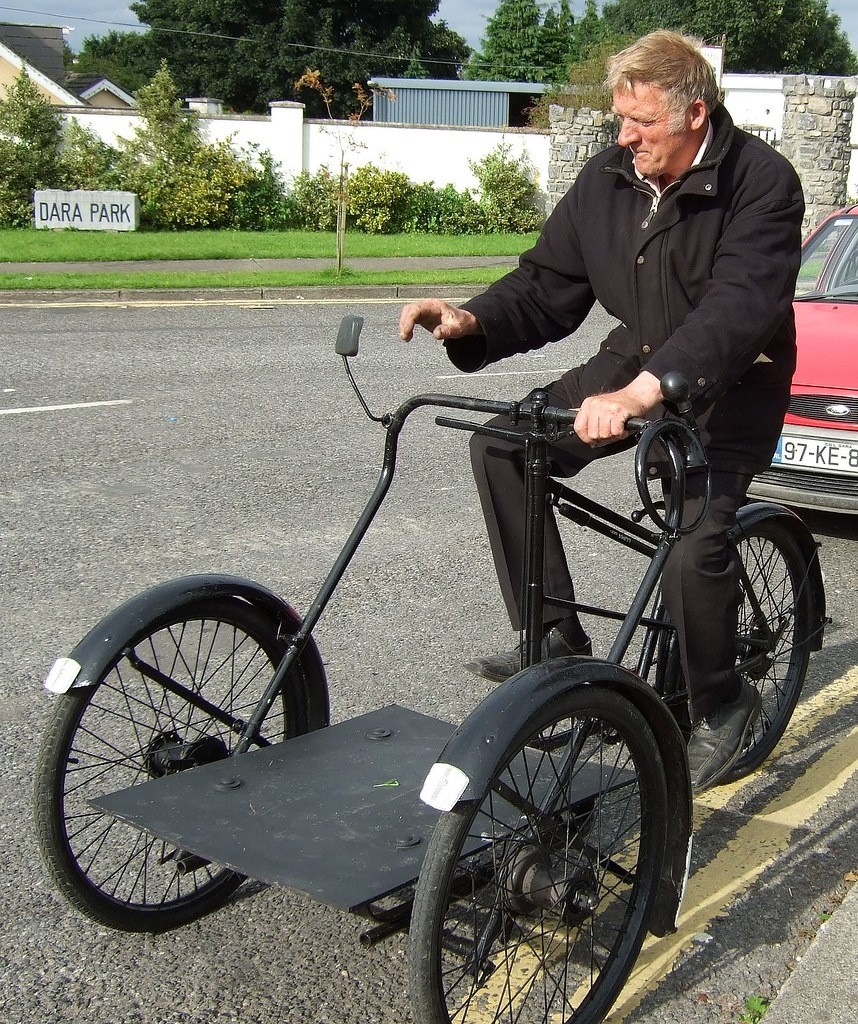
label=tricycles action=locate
[32,315,835,1024]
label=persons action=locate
[400,27,805,792]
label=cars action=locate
[747,200,858,516]
